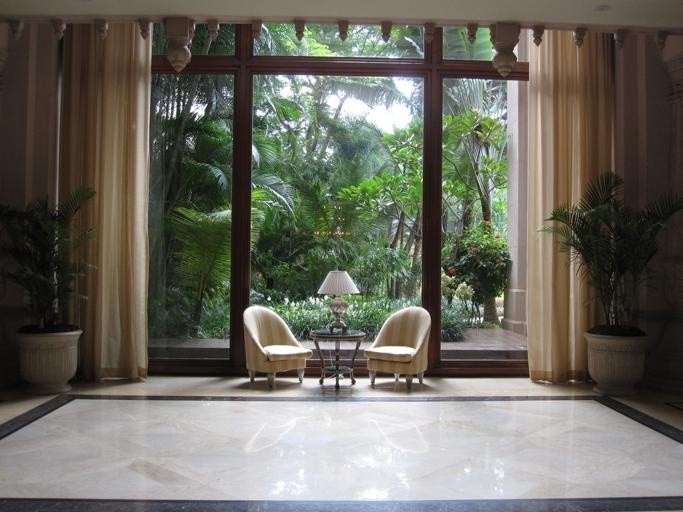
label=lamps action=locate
[315,270,362,335]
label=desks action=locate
[308,329,366,390]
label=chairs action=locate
[241,304,313,391]
[362,305,432,391]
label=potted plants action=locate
[1,184,101,395]
[533,171,682,399]
